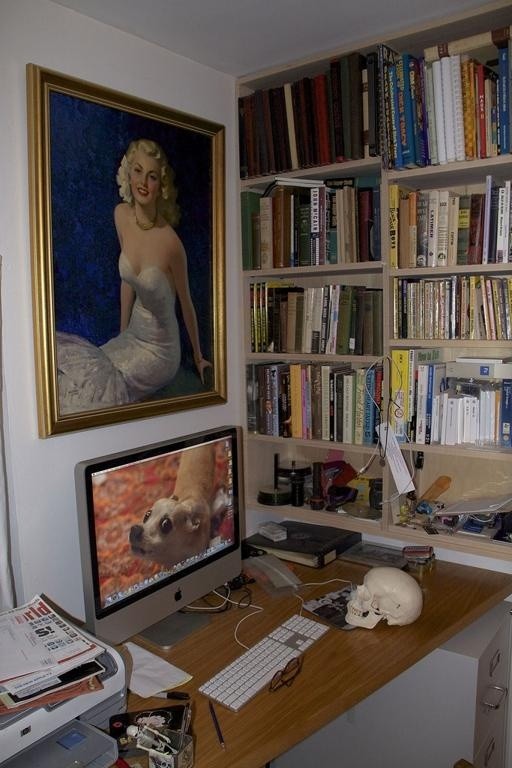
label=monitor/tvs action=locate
[73,425,247,650]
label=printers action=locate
[0,616,128,768]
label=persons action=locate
[56,137,215,417]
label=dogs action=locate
[129,442,217,566]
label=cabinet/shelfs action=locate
[233,1,512,560]
[269,601,512,768]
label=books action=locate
[0,592,107,714]
[390,349,511,450]
[246,519,363,568]
[377,26,509,172]
[241,176,382,270]
[246,360,384,446]
[248,279,382,356]
[394,275,511,339]
[240,51,379,181]
[388,174,511,268]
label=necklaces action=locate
[132,206,160,230]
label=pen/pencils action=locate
[115,756,131,768]
[153,691,190,700]
[210,699,226,751]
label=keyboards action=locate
[197,614,331,713]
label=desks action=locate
[110,555,512,768]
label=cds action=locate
[257,486,291,506]
[278,460,312,478]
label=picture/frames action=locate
[338,541,408,570]
[26,61,230,441]
